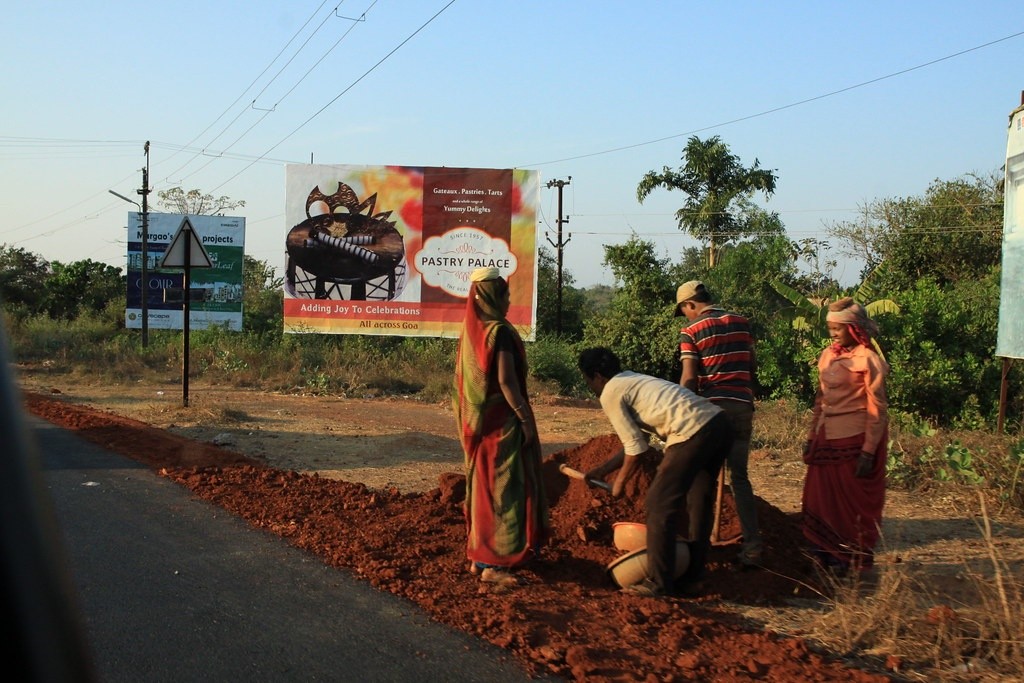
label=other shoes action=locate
[618,583,668,597]
[816,554,841,566]
[674,585,704,596]
[862,549,874,565]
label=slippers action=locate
[480,572,521,584]
[469,567,478,576]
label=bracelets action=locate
[861,454,873,460]
[514,404,529,423]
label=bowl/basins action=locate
[612,523,647,551]
[605,544,649,589]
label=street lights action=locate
[108,188,148,351]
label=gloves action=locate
[854,450,876,479]
[803,440,814,463]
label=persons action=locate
[799,298,889,574]
[452,269,544,587]
[576,347,728,594]
[673,280,762,561]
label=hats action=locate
[673,279,706,317]
